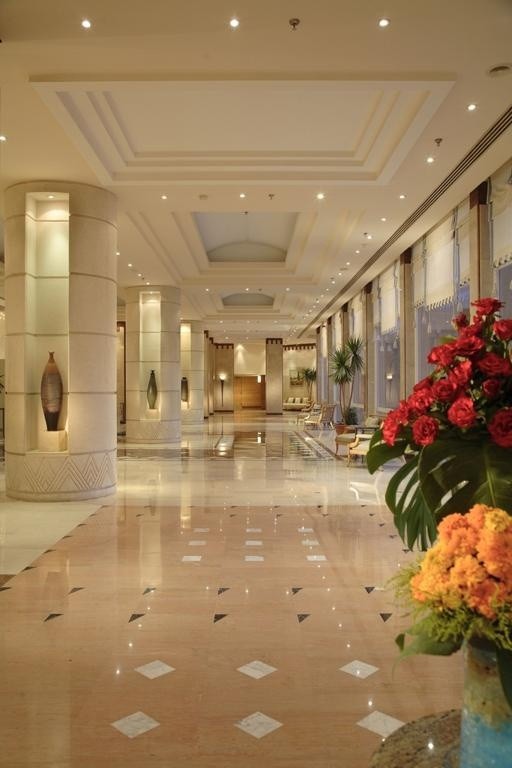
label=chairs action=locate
[304,403,336,432]
[334,414,382,466]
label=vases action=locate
[458,641,512,767]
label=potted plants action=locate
[327,336,366,434]
